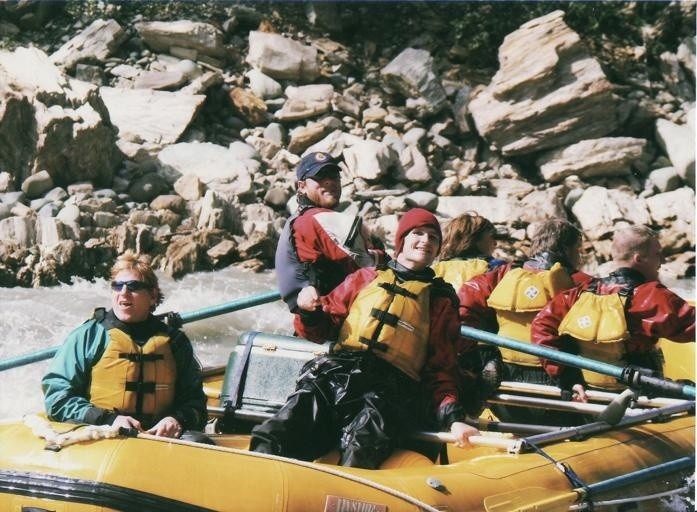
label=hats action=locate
[296,152,342,182]
[395,208,442,257]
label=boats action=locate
[2,298,695,512]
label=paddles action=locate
[483,456,695,511]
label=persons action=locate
[40,253,215,447]
[274,151,393,344]
[456,217,592,387]
[429,210,511,294]
[531,225,696,404]
[250,207,481,468]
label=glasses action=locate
[109,279,153,292]
[302,166,340,182]
